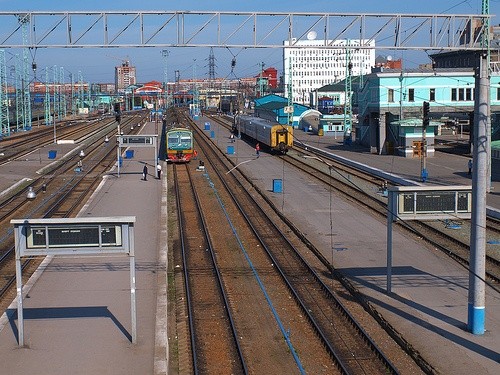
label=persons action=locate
[255,142,261,154]
[468,159,472,175]
[156,163,162,179]
[143,163,148,181]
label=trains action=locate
[233,112,294,155]
[98,103,108,114]
[165,109,193,164]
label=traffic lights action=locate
[423,121,429,125]
[114,101,120,112]
[423,100,430,118]
[116,115,120,121]
